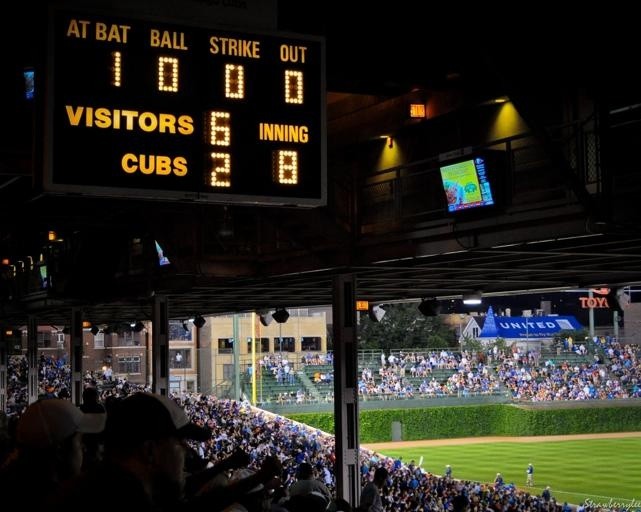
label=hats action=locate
[20,399,106,441]
[117,391,212,441]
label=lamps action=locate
[606,286,630,313]
[462,293,482,306]
[61,306,290,336]
[417,296,442,316]
[368,302,385,321]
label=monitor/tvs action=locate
[436,150,511,219]
[41,10,328,210]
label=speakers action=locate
[193,317,205,328]
[419,298,442,317]
[90,327,99,335]
[272,309,289,323]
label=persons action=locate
[0,353,639,512]
[262,334,641,403]
